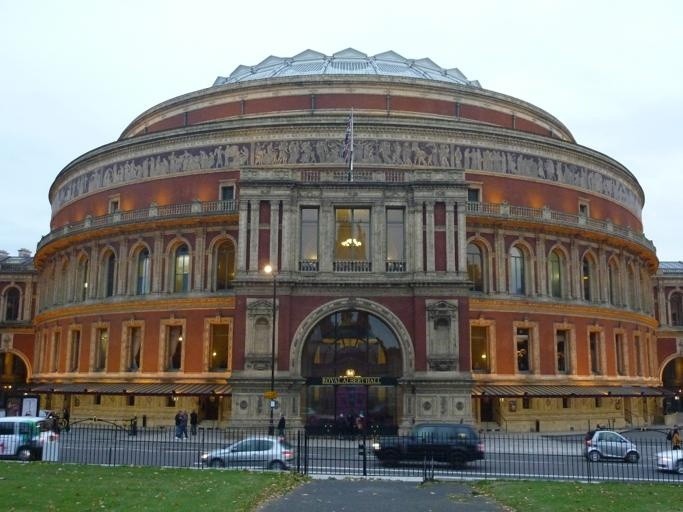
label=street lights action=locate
[264,265,276,436]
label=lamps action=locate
[341,238,361,248]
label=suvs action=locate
[0,416,60,461]
[371,424,485,468]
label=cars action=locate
[656,450,683,474]
[584,431,640,463]
[201,437,294,471]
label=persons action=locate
[667,422,682,450]
[337,410,365,437]
[278,412,286,438]
[173,409,199,440]
[62,409,72,434]
